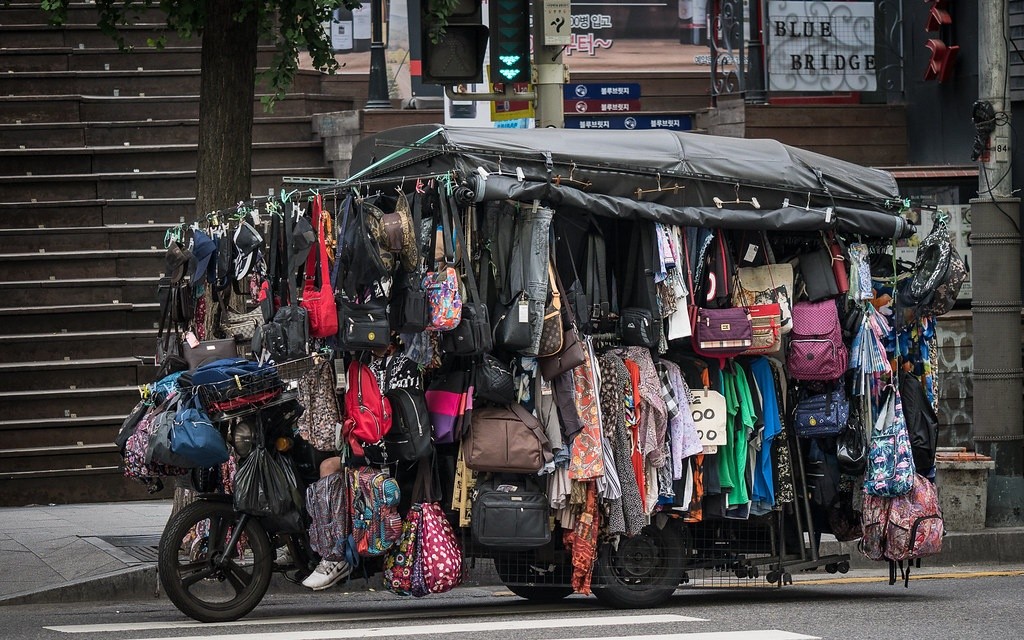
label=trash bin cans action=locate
[936,449,995,533]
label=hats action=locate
[165,189,419,283]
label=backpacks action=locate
[788,300,848,379]
[899,210,967,317]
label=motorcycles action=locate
[157,353,689,622]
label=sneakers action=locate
[302,559,353,591]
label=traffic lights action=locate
[419,0,489,84]
[489,0,531,83]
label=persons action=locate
[302,457,353,591]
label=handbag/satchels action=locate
[799,247,839,302]
[689,306,752,358]
[735,285,793,336]
[539,328,585,382]
[798,382,945,560]
[732,262,794,304]
[739,305,781,355]
[541,296,563,356]
[617,309,660,347]
[119,265,552,594]
[561,282,589,329]
